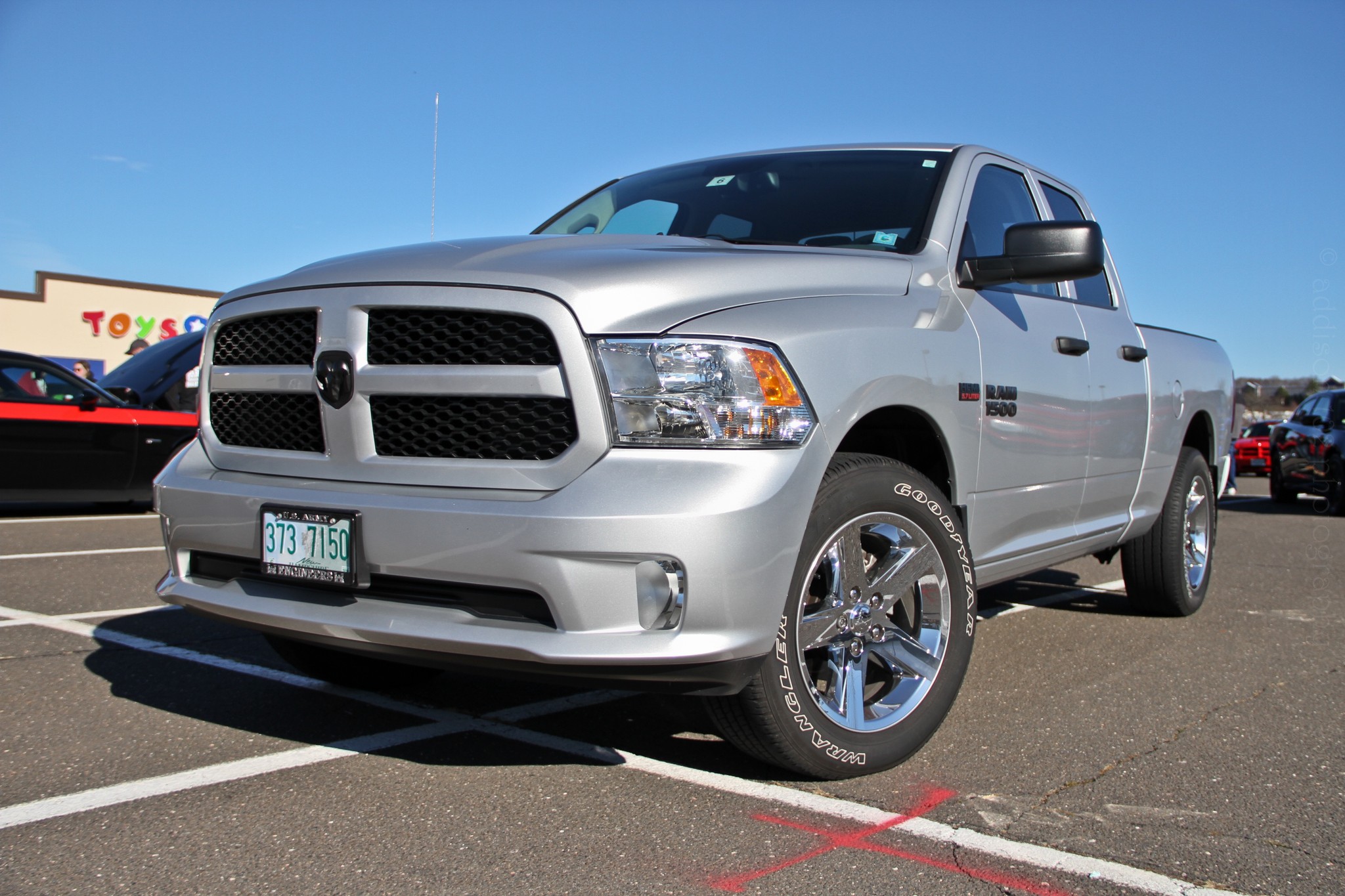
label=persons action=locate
[18,367,48,397]
[73,360,90,379]
[124,339,148,357]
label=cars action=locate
[1270,389,1345,513]
[1235,417,1289,476]
[0,329,207,513]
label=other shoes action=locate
[1224,487,1236,496]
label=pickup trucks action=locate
[154,141,1234,781]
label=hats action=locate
[125,340,149,354]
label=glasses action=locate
[73,368,80,372]
